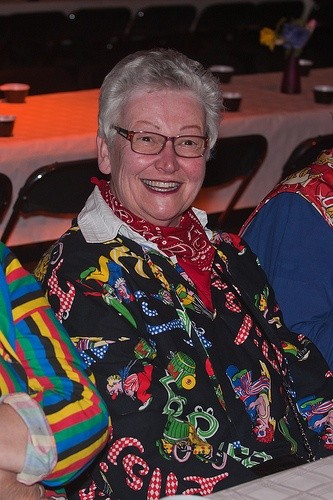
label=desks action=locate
[0,67,333,246]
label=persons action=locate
[0,239,111,500]
[0,49,333,500]
[236,149,333,374]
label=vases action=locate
[281,53,301,94]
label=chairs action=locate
[0,157,111,245]
[202,134,269,229]
[0,0,333,98]
[278,134,333,181]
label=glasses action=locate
[112,125,209,158]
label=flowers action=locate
[260,0,316,55]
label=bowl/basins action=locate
[0,115,16,137]
[2,83,30,103]
[313,85,333,104]
[210,65,234,84]
[222,91,243,110]
[299,59,312,75]
